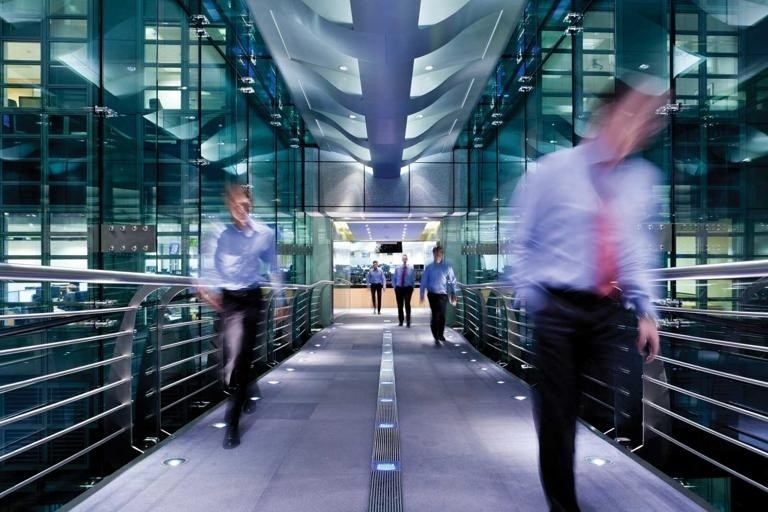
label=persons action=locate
[420,246,457,348]
[391,253,415,329]
[193,184,292,450]
[366,261,387,315]
[504,70,672,512]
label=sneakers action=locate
[435,335,445,348]
[407,322,410,328]
[398,320,403,327]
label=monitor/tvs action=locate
[376,241,402,253]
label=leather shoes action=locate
[222,424,242,450]
[243,400,257,414]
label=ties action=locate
[400,265,406,287]
[595,195,618,295]
